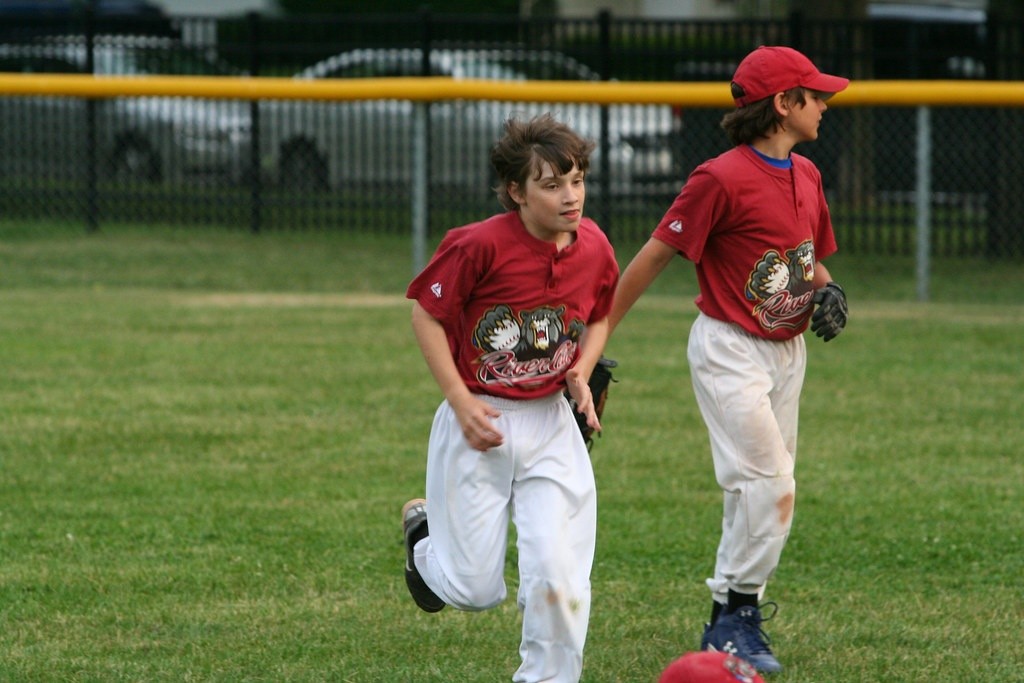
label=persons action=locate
[402,114,620,683]
[608,46,850,674]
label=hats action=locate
[658,652,765,683]
[730,46,849,107]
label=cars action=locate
[258,42,685,202]
[0,32,256,187]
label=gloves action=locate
[810,282,848,342]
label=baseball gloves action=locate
[565,354,614,449]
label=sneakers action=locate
[401,498,446,613]
[701,602,781,671]
